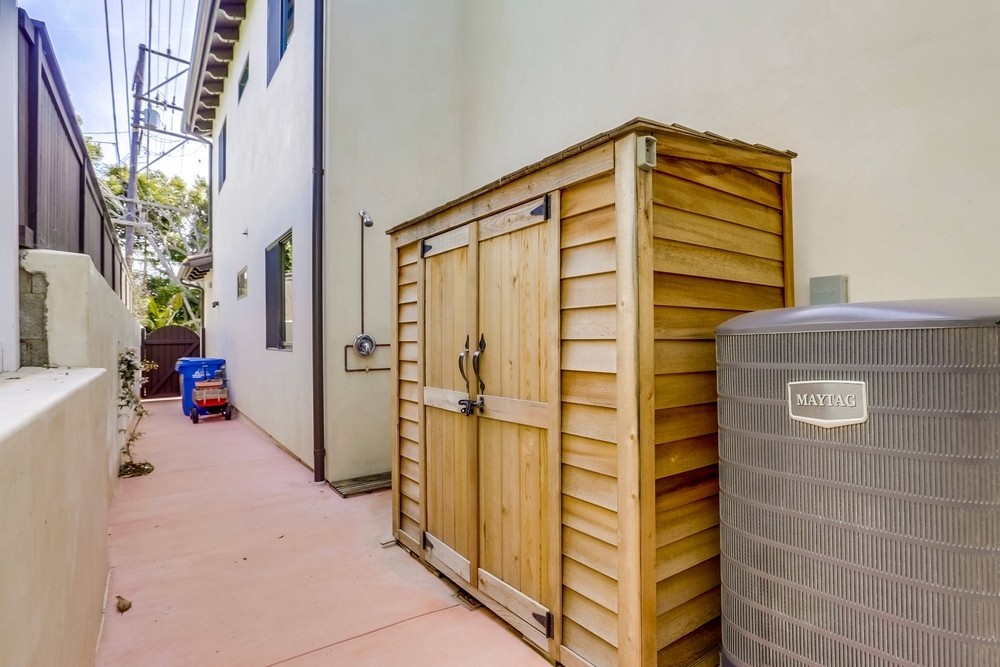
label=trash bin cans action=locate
[174,356,227,416]
